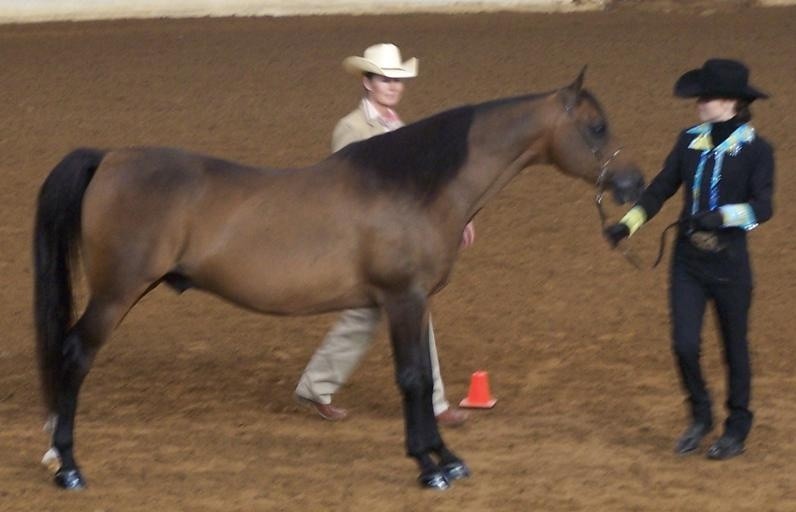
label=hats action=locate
[342,42,418,79]
[673,59,768,99]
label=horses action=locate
[30,62,647,493]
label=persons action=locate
[295,44,478,425]
[602,57,777,460]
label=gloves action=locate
[601,223,629,250]
[692,207,722,230]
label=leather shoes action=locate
[675,417,716,456]
[292,390,348,420]
[705,434,748,461]
[434,408,469,427]
